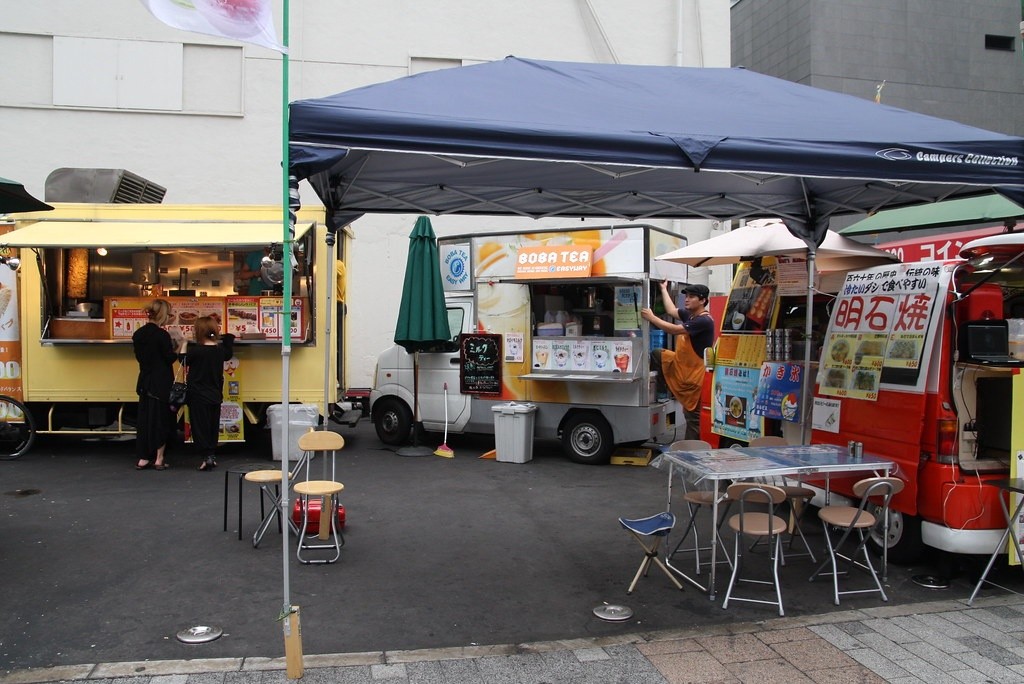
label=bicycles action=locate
[0,394,35,461]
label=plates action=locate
[824,338,919,392]
[728,396,743,418]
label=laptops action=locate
[965,320,1024,365]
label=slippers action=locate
[135,459,154,470]
[154,459,173,469]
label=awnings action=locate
[496,275,641,286]
[0,220,315,247]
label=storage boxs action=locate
[537,323,564,336]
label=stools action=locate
[618,511,685,595]
[224,464,281,540]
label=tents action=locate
[284,54,1023,633]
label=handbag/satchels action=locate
[169,355,192,406]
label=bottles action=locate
[544,309,571,325]
[847,440,855,457]
[855,442,863,458]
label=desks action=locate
[664,444,896,601]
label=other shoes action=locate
[207,459,217,468]
[656,391,668,403]
[195,460,208,472]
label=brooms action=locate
[433,382,454,459]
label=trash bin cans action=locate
[269,404,319,461]
[491,402,537,464]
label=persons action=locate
[179,317,236,470]
[642,278,715,442]
[131,299,185,469]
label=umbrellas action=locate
[394,215,450,458]
[652,217,898,270]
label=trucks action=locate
[370,225,689,466]
[0,203,355,455]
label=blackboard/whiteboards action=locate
[460,333,503,396]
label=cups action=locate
[534,349,629,372]
[732,311,744,329]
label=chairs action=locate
[669,441,734,575]
[722,482,786,617]
[808,477,904,605]
[748,436,818,566]
[244,427,314,548]
[294,431,345,564]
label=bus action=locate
[709,252,1024,568]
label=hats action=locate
[681,285,710,298]
